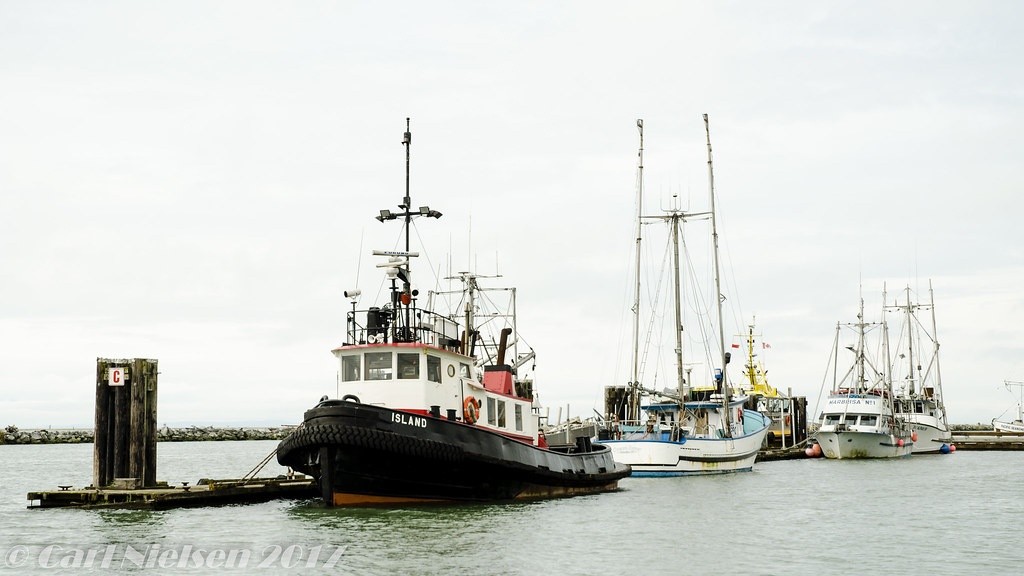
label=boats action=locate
[692,315,821,462]
[276,118,632,509]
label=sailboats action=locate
[873,279,955,454]
[809,273,918,456]
[591,113,773,478]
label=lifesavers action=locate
[738,408,742,421]
[786,416,790,427]
[464,397,480,424]
